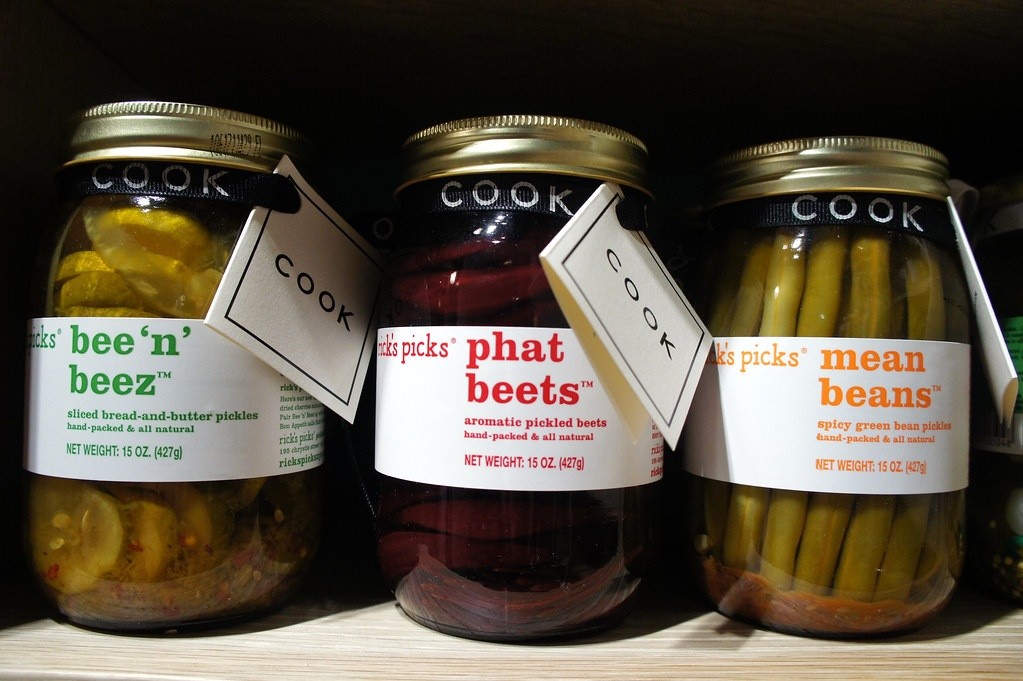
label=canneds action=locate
[370,114,648,641]
[695,137,970,631]
[28,100,324,632]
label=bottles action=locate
[366,119,663,633]
[19,98,325,627]
[682,134,981,631]
